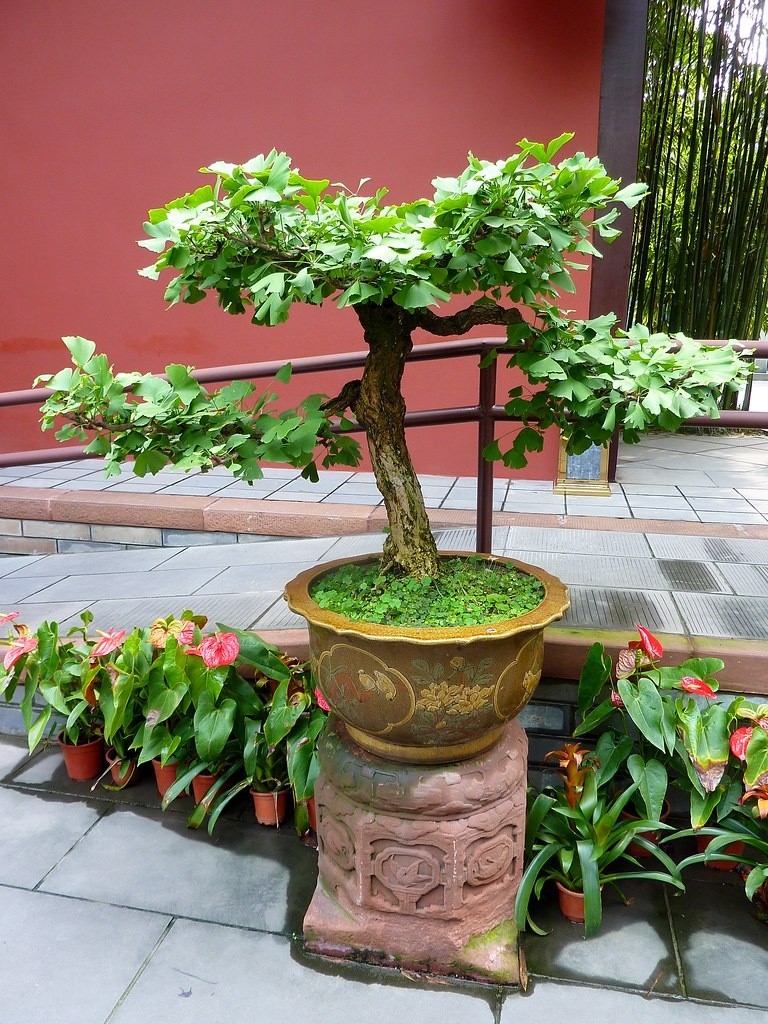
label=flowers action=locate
[281,669,334,846]
[237,650,311,794]
[127,607,209,766]
[0,610,107,756]
[570,621,724,839]
[692,694,768,836]
[80,621,158,792]
[160,618,293,829]
[512,743,686,942]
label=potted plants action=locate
[32,133,756,764]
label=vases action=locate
[150,755,198,797]
[106,744,149,787]
[698,816,746,870]
[306,794,318,833]
[188,768,231,811]
[614,788,670,856]
[250,783,290,826]
[52,723,104,781]
[555,870,604,923]
